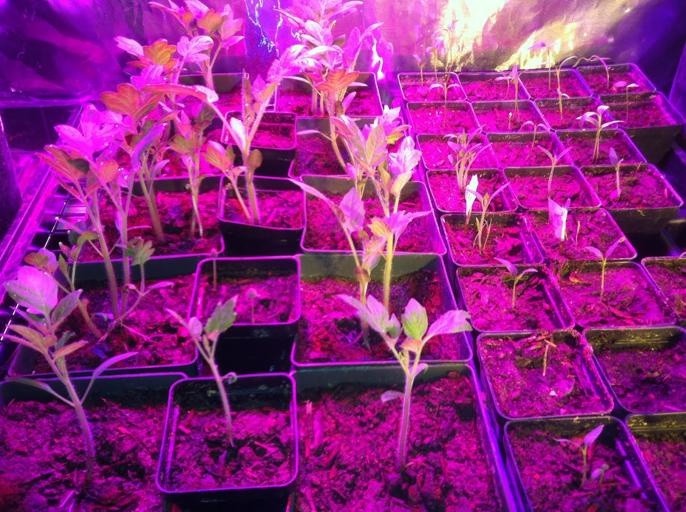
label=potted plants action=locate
[2,6,686,507]
